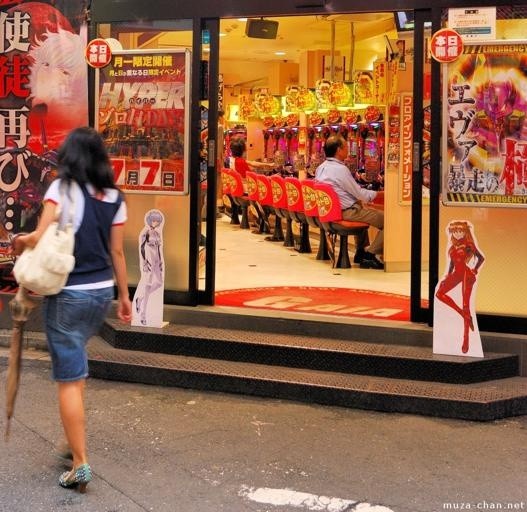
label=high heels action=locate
[58,464,92,493]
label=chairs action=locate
[211,169,378,271]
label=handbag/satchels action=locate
[11,181,80,296]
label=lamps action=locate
[245,15,278,39]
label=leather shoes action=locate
[354,248,384,269]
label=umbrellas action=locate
[7,231,37,440]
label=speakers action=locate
[245,18,279,39]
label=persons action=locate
[13,127,132,493]
[216,111,225,218]
[313,136,384,270]
[229,141,280,179]
[389,40,404,62]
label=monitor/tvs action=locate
[393,10,432,32]
